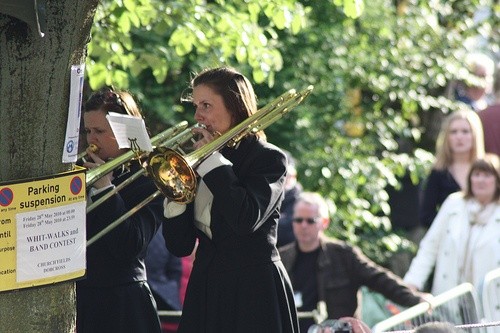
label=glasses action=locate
[291,218,316,224]
[307,324,337,333]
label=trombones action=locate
[75,121,189,187]
[86,84,314,249]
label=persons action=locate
[71,89,166,333]
[448,54,500,155]
[162,69,300,333]
[421,108,486,229]
[143,178,436,333]
[403,156,500,333]
